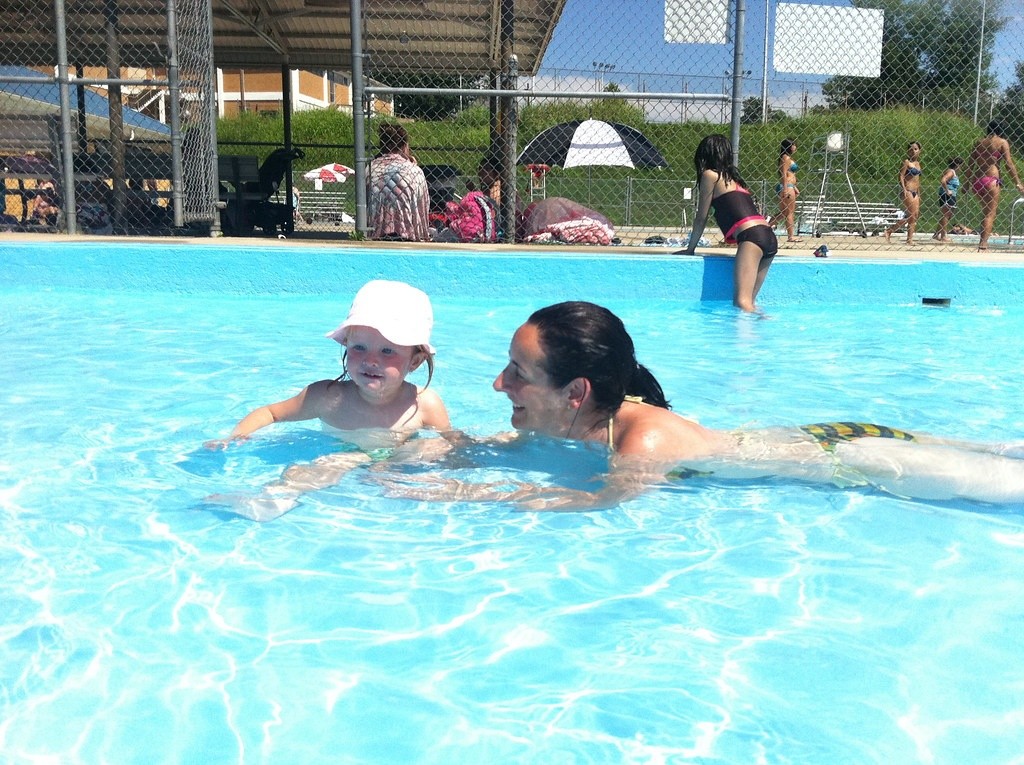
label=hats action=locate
[325,280,437,357]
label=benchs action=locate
[795,200,906,225]
[0,113,97,230]
[73,154,267,236]
[270,191,347,214]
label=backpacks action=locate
[455,191,501,244]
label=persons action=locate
[232,280,453,431]
[494,302,734,458]
[181,127,202,155]
[33,178,55,215]
[365,121,432,242]
[770,137,803,242]
[292,183,303,223]
[931,158,965,240]
[479,157,501,206]
[146,179,158,191]
[884,142,922,246]
[953,223,998,236]
[962,120,1024,249]
[673,134,778,309]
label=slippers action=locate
[787,238,804,242]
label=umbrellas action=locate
[304,162,355,184]
[516,108,668,204]
